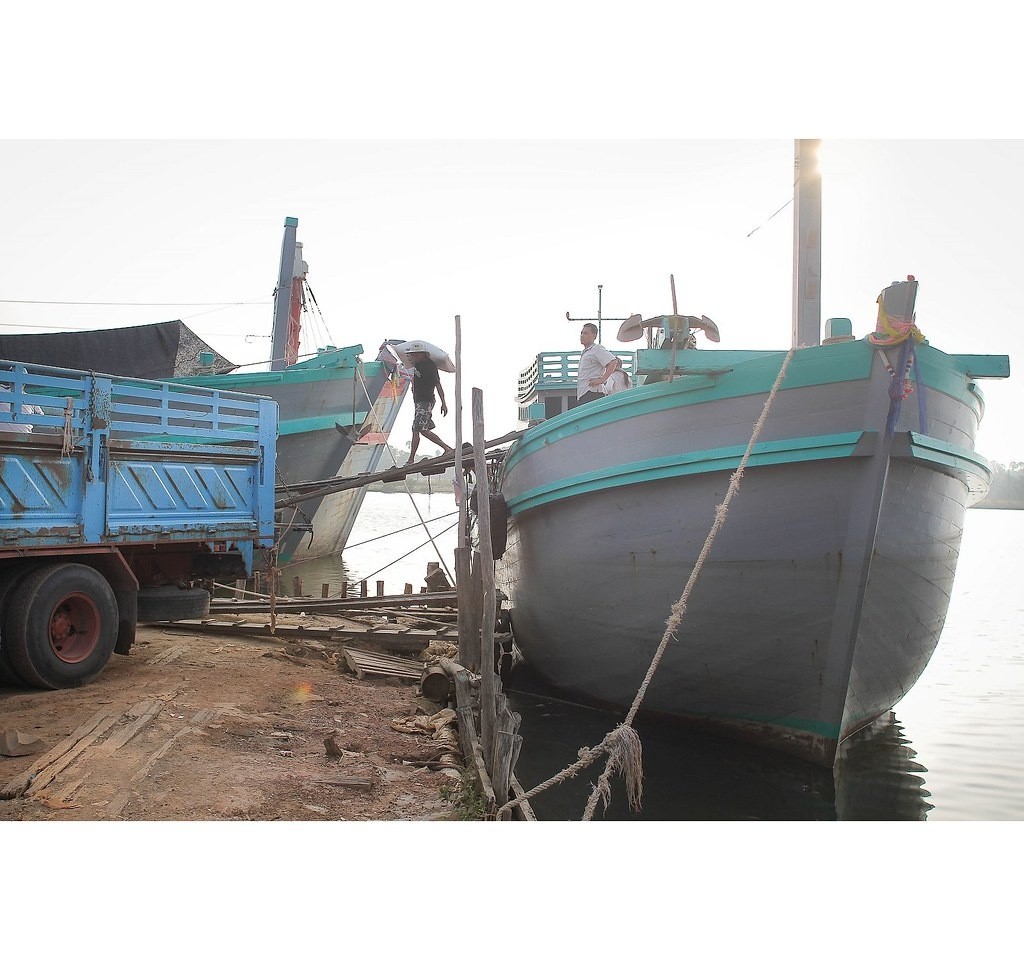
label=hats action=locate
[405,342,430,354]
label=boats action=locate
[496,140,1011,766]
[1,212,452,573]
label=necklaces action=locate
[581,350,588,356]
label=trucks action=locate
[1,360,283,691]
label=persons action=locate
[577,323,617,405]
[403,344,453,467]
[607,357,632,395]
